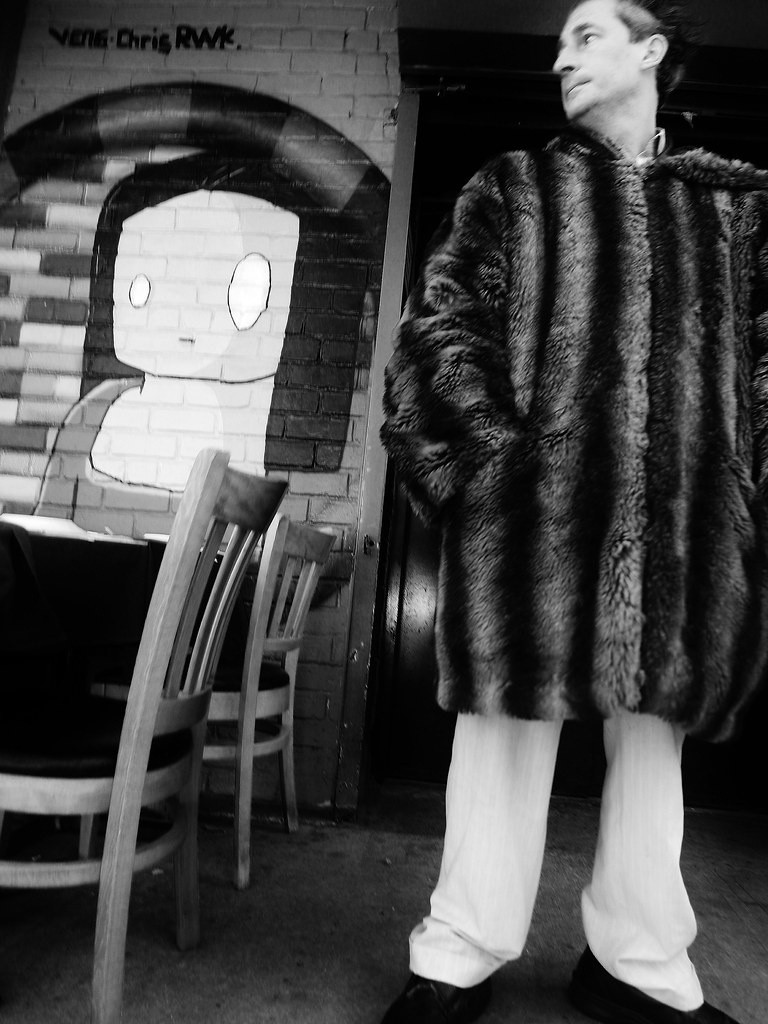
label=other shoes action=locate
[565,943,739,1024]
[379,975,496,1024]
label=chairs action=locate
[2,449,290,1024]
[79,512,336,890]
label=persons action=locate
[385,0,768,1023]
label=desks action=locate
[0,520,256,860]
